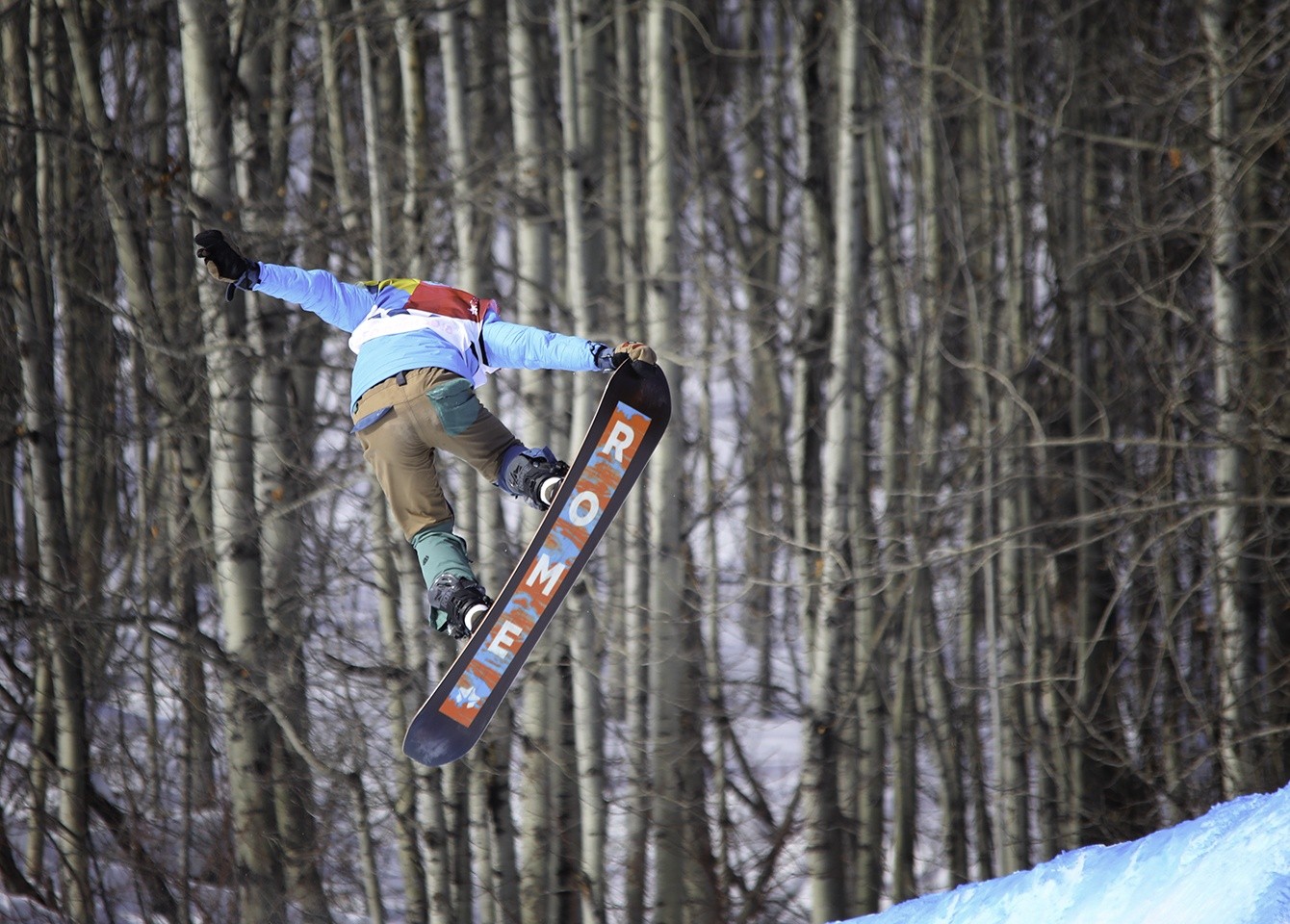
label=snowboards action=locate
[401,354,675,768]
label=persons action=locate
[193,229,657,640]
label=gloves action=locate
[610,340,658,372]
[193,229,257,302]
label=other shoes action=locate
[463,601,488,633]
[536,477,562,507]
